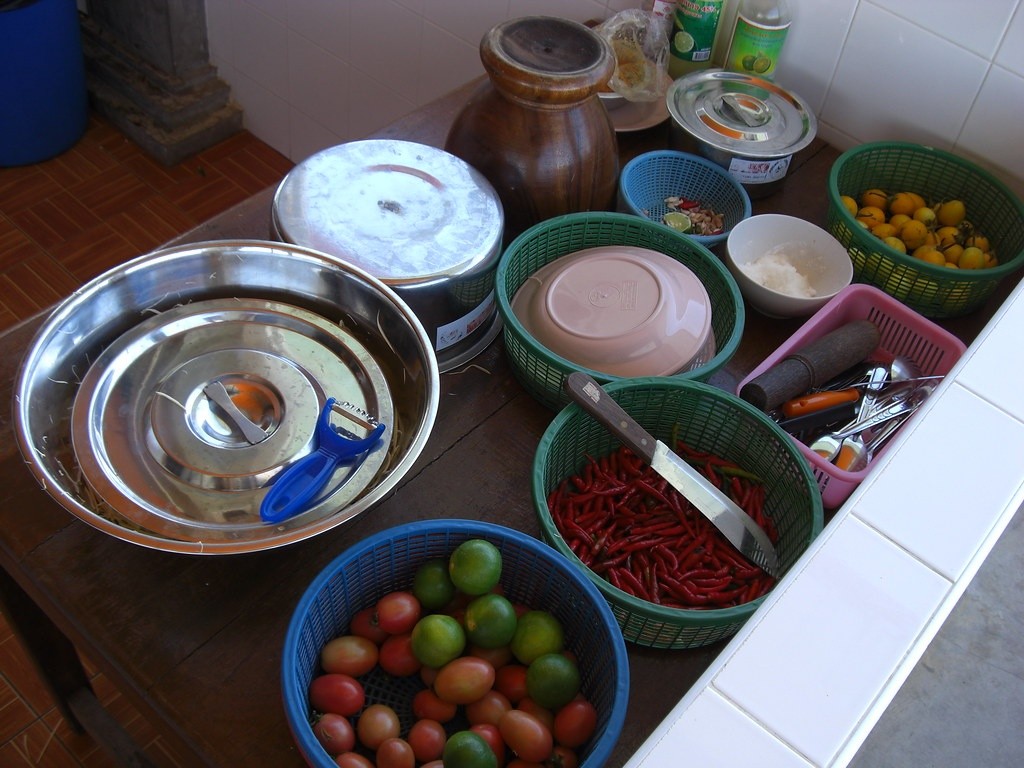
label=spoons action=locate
[769,334,945,483]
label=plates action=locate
[509,245,716,378]
[613,57,674,132]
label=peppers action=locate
[549,419,777,613]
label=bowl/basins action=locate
[597,64,649,111]
[270,138,504,374]
[10,240,441,555]
[666,69,818,200]
[725,214,853,319]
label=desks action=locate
[0,20,1024,768]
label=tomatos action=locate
[841,187,998,316]
[310,590,596,768]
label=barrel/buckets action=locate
[0,0,87,168]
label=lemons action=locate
[408,538,581,768]
[742,55,772,73]
[675,31,694,52]
[663,212,692,234]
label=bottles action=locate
[723,0,792,81]
[668,0,725,78]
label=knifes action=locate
[567,372,786,578]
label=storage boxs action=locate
[733,282,968,510]
